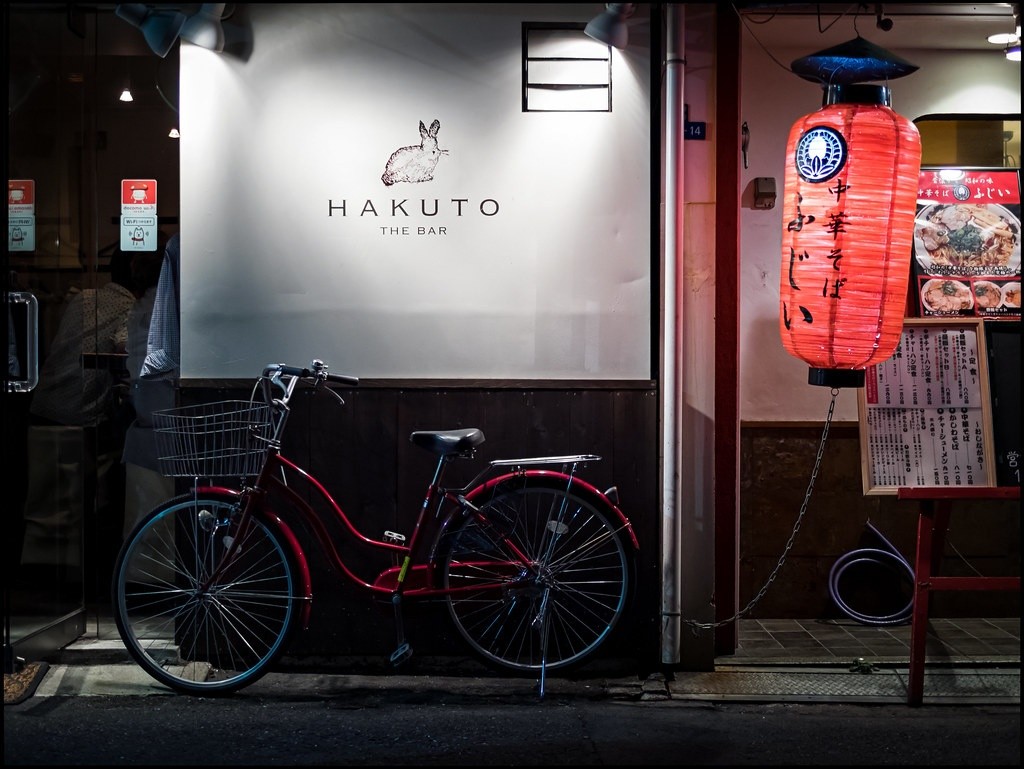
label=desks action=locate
[894,486,1024,712]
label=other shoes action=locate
[125,583,175,614]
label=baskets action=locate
[152,400,271,476]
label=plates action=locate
[974,281,1005,308]
[914,204,1022,278]
[1000,282,1021,307]
[921,278,974,313]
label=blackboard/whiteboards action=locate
[984,315,1024,498]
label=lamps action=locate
[582,2,636,52]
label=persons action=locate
[29,227,169,455]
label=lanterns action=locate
[777,82,922,388]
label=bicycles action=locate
[111,358,640,697]
